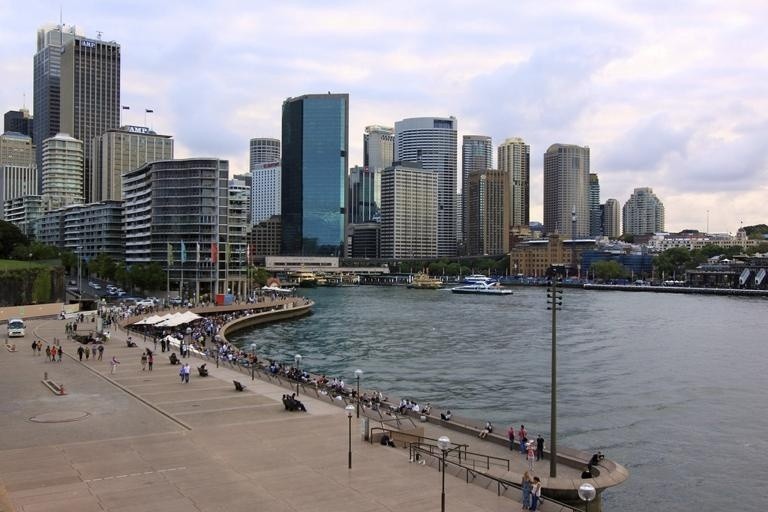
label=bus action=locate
[6,317,27,338]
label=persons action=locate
[588,275,767,292]
[527,476,541,512]
[520,471,532,510]
[315,374,546,474]
[30,287,314,386]
[587,452,601,472]
[286,395,307,412]
[282,394,287,400]
[291,393,297,401]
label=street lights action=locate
[577,483,598,512]
[355,368,364,419]
[250,344,257,380]
[344,404,356,469]
[543,264,564,480]
[294,353,303,395]
[436,435,450,512]
[111,305,223,369]
[75,245,83,295]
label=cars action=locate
[70,278,193,317]
[499,273,685,288]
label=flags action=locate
[180,239,187,265]
[196,243,201,263]
[166,242,174,267]
[238,242,244,261]
[246,243,255,260]
[210,242,217,264]
[224,242,232,264]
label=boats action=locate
[450,276,513,297]
[405,270,444,290]
[359,274,408,287]
[262,285,292,295]
[460,273,498,284]
